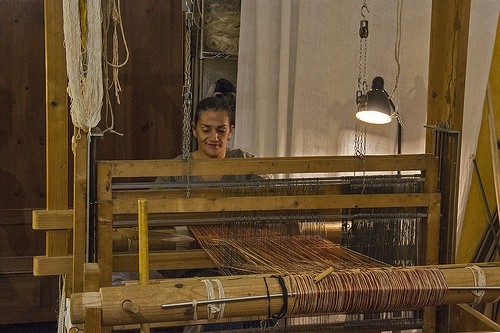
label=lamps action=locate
[355,77,401,176]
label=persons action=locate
[149,98,275,216]
